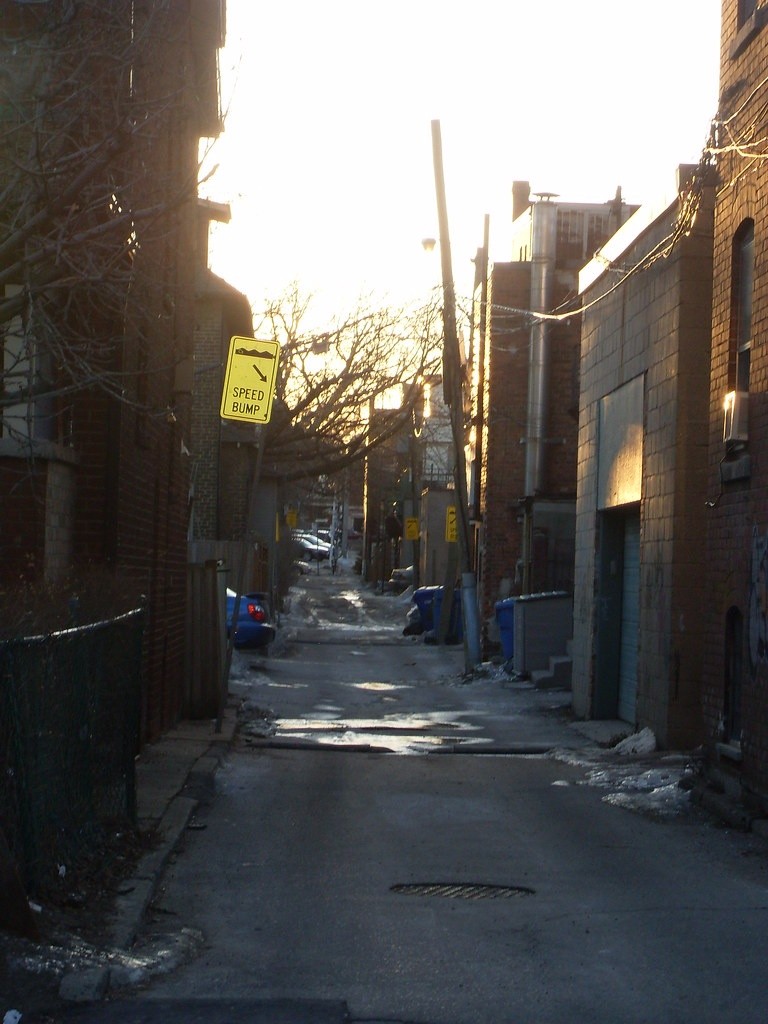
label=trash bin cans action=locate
[411,586,445,631]
[432,589,463,641]
[495,596,514,660]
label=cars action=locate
[291,529,361,562]
[227,588,275,650]
